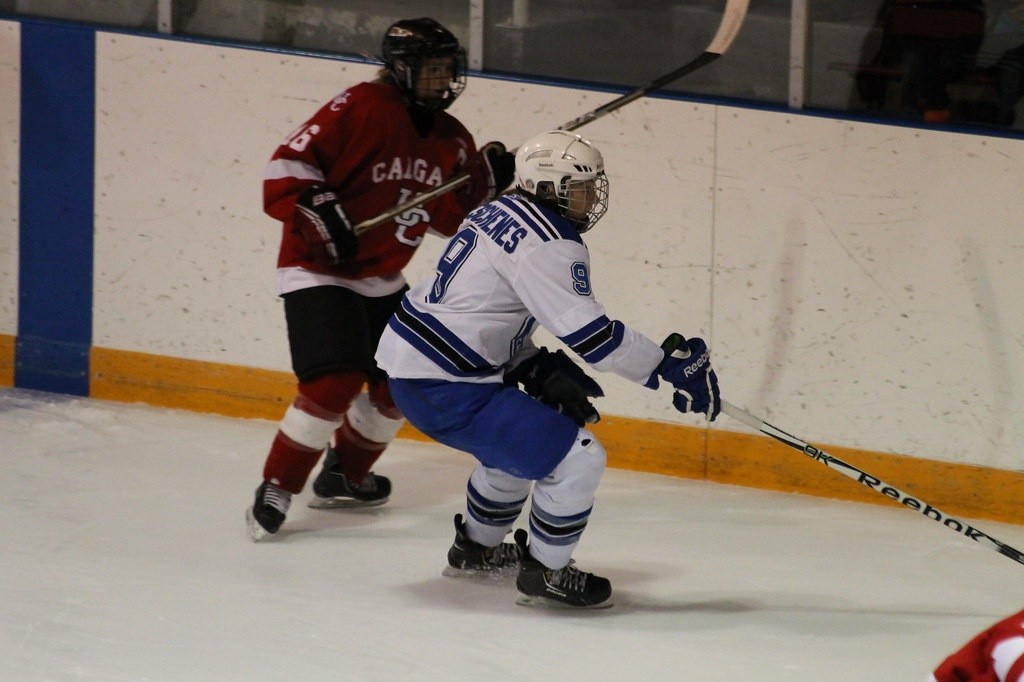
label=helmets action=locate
[382,17,467,110]
[515,130,604,231]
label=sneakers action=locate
[514,529,614,609]
[307,441,392,508]
[442,513,520,577]
[245,479,292,541]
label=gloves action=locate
[660,332,721,423]
[525,347,605,428]
[292,184,358,265]
[490,152,515,198]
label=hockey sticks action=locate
[716,398,1024,566]
[350,0,751,240]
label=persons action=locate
[374,130,720,607]
[242,16,515,544]
[852,0,1024,133]
